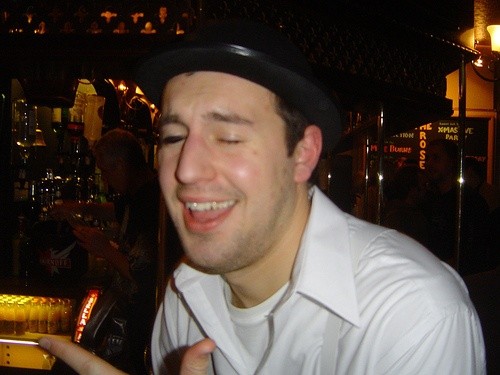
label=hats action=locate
[135,21,344,160]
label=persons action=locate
[333,157,500,271]
[69,129,179,292]
[40,21,486,375]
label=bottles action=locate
[31,176,62,214]
[0,294,73,335]
[20,103,38,144]
[77,164,94,203]
